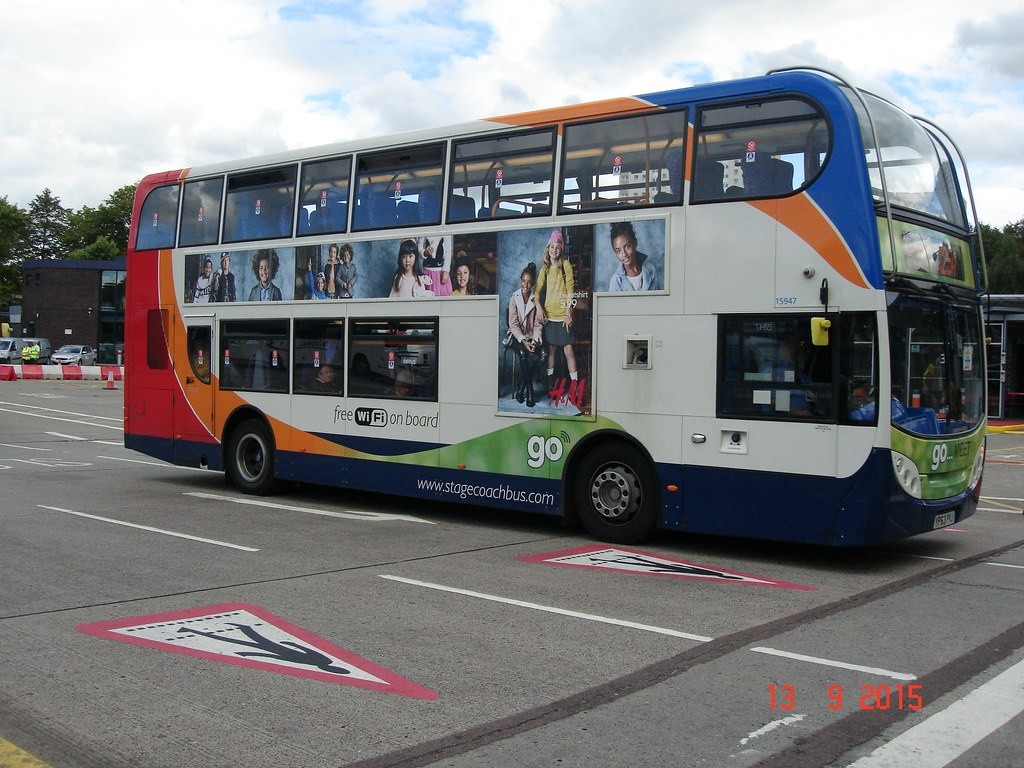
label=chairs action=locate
[134,145,796,254]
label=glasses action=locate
[320,372,335,376]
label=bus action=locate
[124,66,991,546]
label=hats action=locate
[547,230,564,252]
[455,251,471,266]
[316,271,326,282]
[204,255,214,266]
[220,252,231,262]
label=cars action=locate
[1,333,98,366]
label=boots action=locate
[516,383,525,403]
[546,374,556,400]
[526,385,535,407]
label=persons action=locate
[922,357,946,409]
[501,230,585,408]
[193,252,236,302]
[307,365,340,392]
[609,221,659,292]
[759,332,816,417]
[21,340,41,365]
[389,235,471,298]
[386,370,416,396]
[248,250,283,301]
[308,243,359,300]
